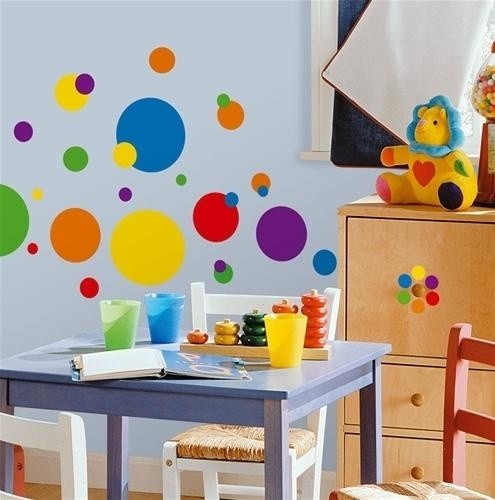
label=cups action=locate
[264,313,308,368]
[99,292,186,351]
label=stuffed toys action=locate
[376,93,479,210]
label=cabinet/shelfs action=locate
[334,193,495,497]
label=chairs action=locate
[161,280,341,500]
[328,323,495,500]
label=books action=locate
[68,347,253,383]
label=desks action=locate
[0,330,392,500]
